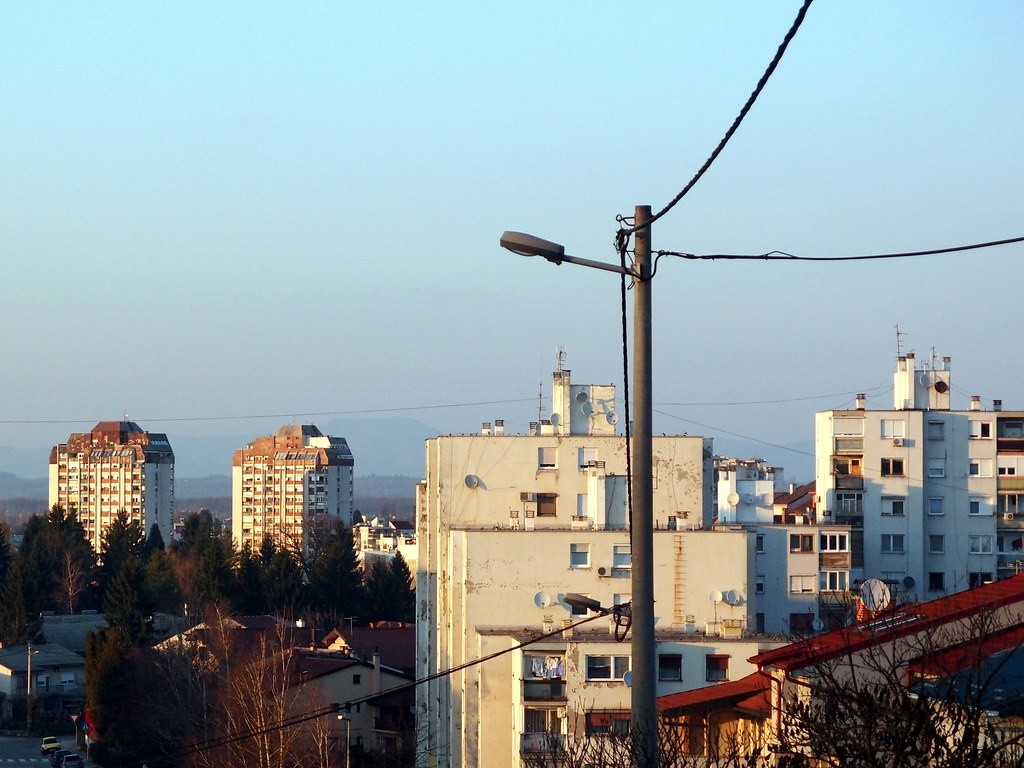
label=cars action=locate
[58,753,85,768]
[39,737,62,755]
[49,748,72,768]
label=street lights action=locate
[500,203,663,768]
[26,644,41,736]
[337,715,352,768]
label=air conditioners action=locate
[520,492,532,501]
[556,707,566,718]
[1003,512,1013,521]
[595,565,611,577]
[892,437,904,447]
